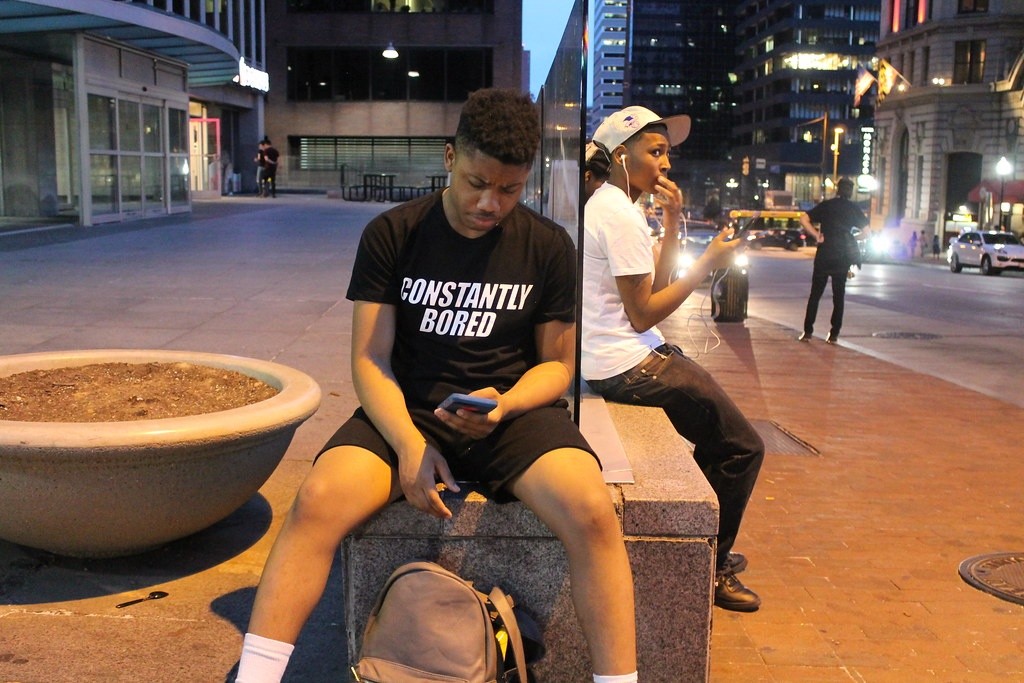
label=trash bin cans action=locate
[232,170,241,194]
[712,266,749,323]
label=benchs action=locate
[342,370,720,683]
[347,184,451,201]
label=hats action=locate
[592,105,692,162]
[585,140,611,174]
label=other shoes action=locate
[826,333,837,344]
[799,332,811,341]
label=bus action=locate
[728,210,809,229]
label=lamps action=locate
[382,0,398,59]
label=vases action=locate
[0,352,320,562]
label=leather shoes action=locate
[724,552,747,572]
[714,564,760,609]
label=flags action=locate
[855,65,875,106]
[877,59,897,105]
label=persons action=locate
[911,231,917,256]
[236,89,638,683]
[932,235,940,259]
[583,105,765,610]
[585,142,611,202]
[174,146,189,175]
[256,139,279,197]
[919,230,926,257]
[799,177,872,344]
[222,150,232,193]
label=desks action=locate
[364,173,394,201]
[426,175,449,192]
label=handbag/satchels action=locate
[848,237,861,268]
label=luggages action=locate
[712,268,749,321]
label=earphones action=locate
[621,154,626,167]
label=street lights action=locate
[832,127,844,187]
[995,156,1011,230]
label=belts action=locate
[587,345,668,392]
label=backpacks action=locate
[350,559,545,683]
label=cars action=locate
[648,208,810,252]
[948,229,1024,276]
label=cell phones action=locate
[735,212,762,239]
[438,393,499,414]
